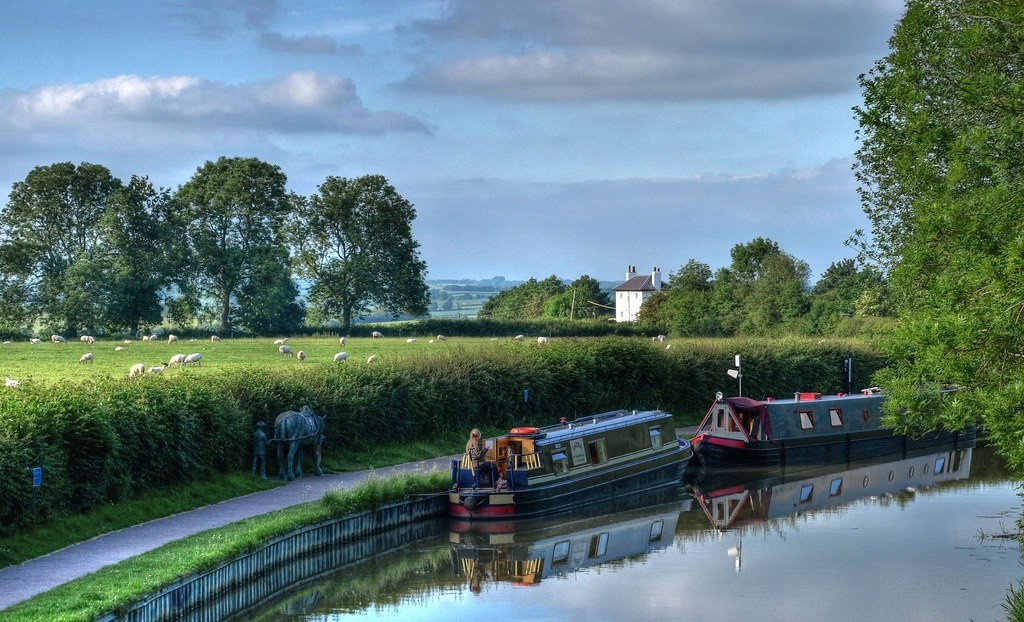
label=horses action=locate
[273,405,328,481]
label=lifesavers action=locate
[511,427,540,435]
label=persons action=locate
[252,421,272,479]
[466,429,508,489]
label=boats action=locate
[447,407,694,521]
[686,437,980,574]
[451,476,694,598]
[688,353,978,468]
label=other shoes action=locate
[501,485,507,488]
[495,478,508,485]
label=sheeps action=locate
[147,361,168,374]
[80,336,96,344]
[2,341,10,344]
[537,336,549,344]
[114,346,124,351]
[123,340,132,343]
[513,334,525,340]
[333,352,348,364]
[29,338,42,344]
[437,334,448,342]
[211,335,223,344]
[5,376,22,386]
[78,352,95,366]
[168,352,203,368]
[143,335,158,342]
[273,338,295,357]
[129,364,145,377]
[428,339,434,344]
[371,331,385,338]
[666,344,672,350]
[366,355,378,364]
[168,334,178,345]
[297,351,305,361]
[652,334,667,342]
[339,336,346,347]
[407,339,417,342]
[51,334,67,344]
[490,337,499,341]
